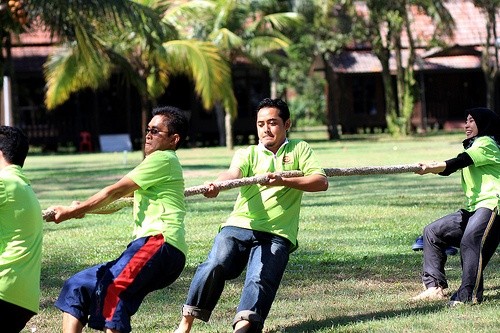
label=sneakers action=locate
[411,287,443,301]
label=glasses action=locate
[145,128,174,136]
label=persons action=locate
[46,106,187,333]
[174,98,328,333]
[0,124,43,333]
[411,108,500,308]
